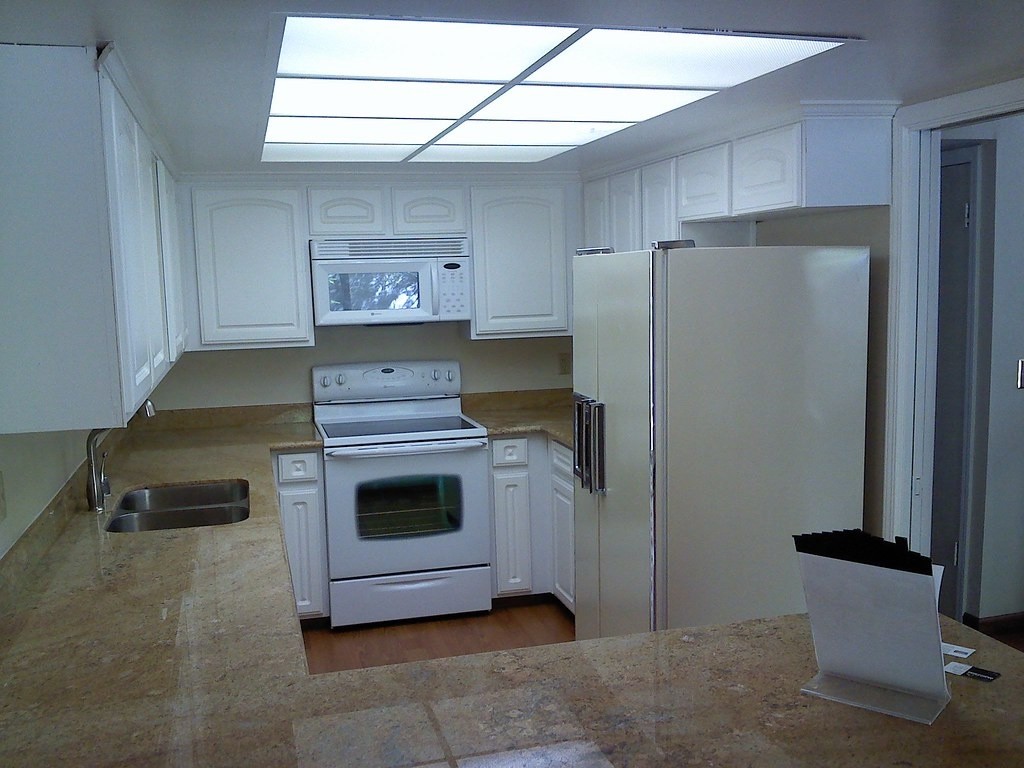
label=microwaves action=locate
[312,257,470,326]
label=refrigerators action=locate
[572,239,869,643]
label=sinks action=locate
[119,479,249,511]
[102,506,250,533]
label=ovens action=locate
[324,438,492,627]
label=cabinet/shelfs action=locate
[490,434,577,612]
[270,448,329,621]
[0,45,893,429]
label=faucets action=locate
[86,399,156,512]
